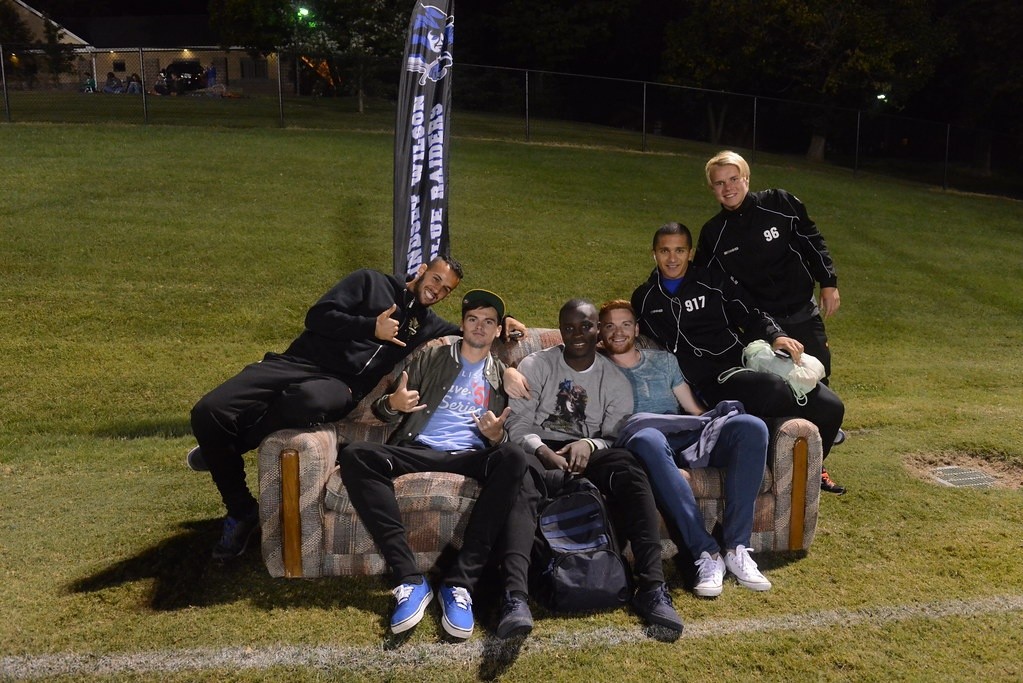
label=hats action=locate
[462,289,506,324]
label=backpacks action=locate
[533,477,627,610]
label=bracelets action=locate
[579,438,596,453]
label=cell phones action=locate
[509,331,524,338]
[774,349,791,358]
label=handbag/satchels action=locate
[742,340,825,398]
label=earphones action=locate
[674,344,677,353]
[653,255,657,262]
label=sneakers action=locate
[721,545,771,590]
[497,598,533,641]
[832,429,844,445]
[212,503,260,558]
[820,465,847,496]
[391,576,434,634]
[694,551,726,596]
[438,584,474,639]
[630,586,684,632]
[187,445,210,471]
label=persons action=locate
[693,150,846,447]
[127,73,145,94]
[80,71,97,94]
[339,289,528,638]
[152,61,217,97]
[504,297,772,596]
[631,223,849,496]
[186,251,528,561]
[496,300,685,638]
[102,71,125,95]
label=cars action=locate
[162,62,206,89]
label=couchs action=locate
[257,327,822,577]
